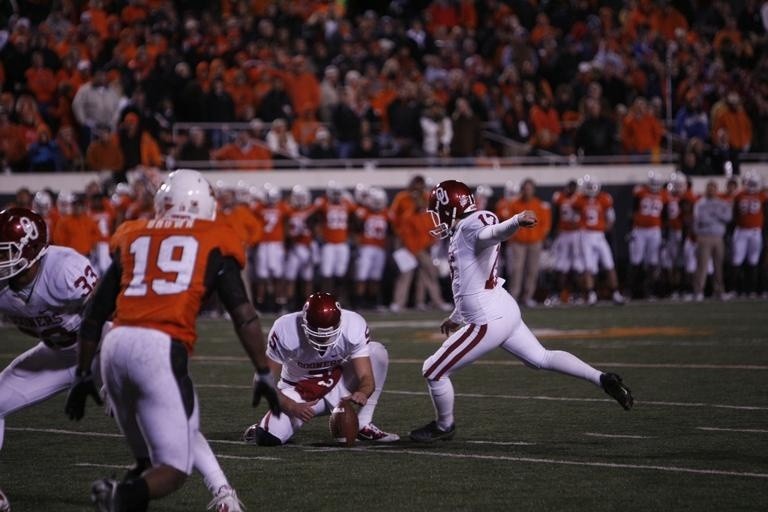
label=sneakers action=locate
[434,302,453,312]
[602,372,634,411]
[355,423,400,445]
[587,290,596,305]
[90,478,122,512]
[717,293,729,304]
[0,493,7,510]
[205,485,246,512]
[747,176,762,195]
[244,423,257,438]
[695,293,704,302]
[524,300,537,308]
[408,421,457,443]
[613,292,625,304]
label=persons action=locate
[499,178,553,308]
[351,185,369,246]
[113,0,230,170]
[496,181,521,220]
[469,186,493,212]
[66,168,282,511]
[5,164,57,243]
[387,176,431,311]
[667,174,767,303]
[659,5,766,172]
[246,291,401,447]
[409,181,634,444]
[48,175,154,254]
[292,182,366,302]
[550,179,586,308]
[202,1,382,166]
[353,187,388,313]
[626,169,665,305]
[1,2,125,171]
[368,3,672,163]
[208,179,259,319]
[561,179,630,307]
[254,188,291,314]
[392,191,454,314]
[285,186,321,300]
[1,206,246,512]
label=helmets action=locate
[368,189,388,210]
[292,185,312,209]
[0,205,51,284]
[326,181,344,205]
[217,181,238,207]
[153,167,219,223]
[426,180,477,240]
[29,191,52,214]
[54,192,77,215]
[355,181,370,204]
[300,292,345,360]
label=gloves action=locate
[250,370,283,420]
[64,369,105,420]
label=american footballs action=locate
[330,399,359,449]
